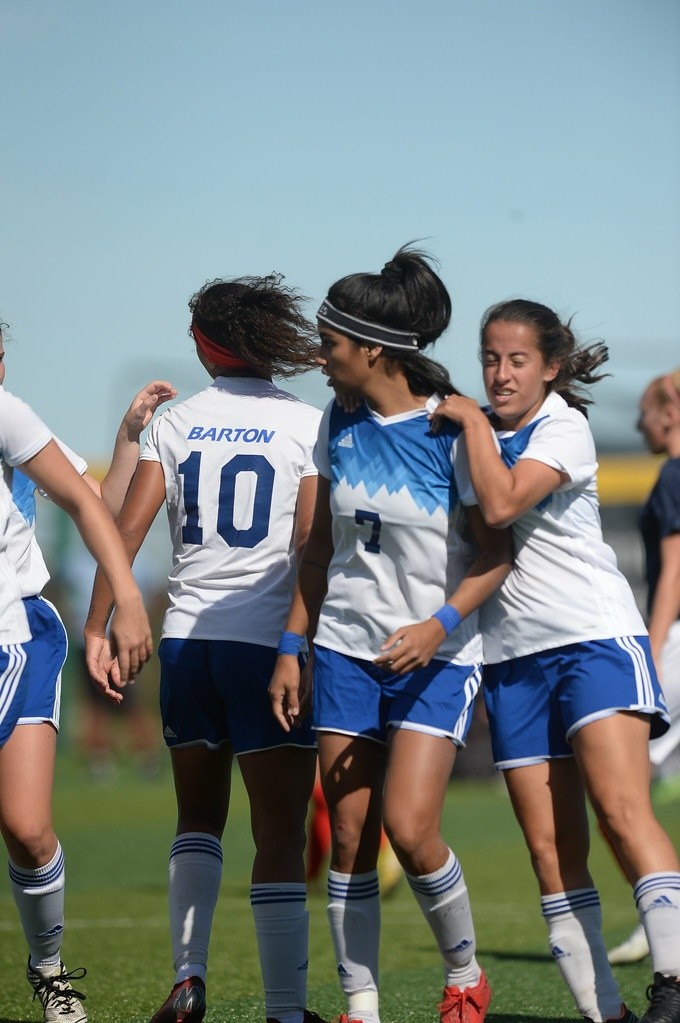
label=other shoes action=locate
[378,847,404,900]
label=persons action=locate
[0,385,154,1023]
[306,755,403,900]
[426,298,680,1023]
[0,320,102,535]
[268,247,516,1023]
[84,273,324,1023]
[606,370,680,965]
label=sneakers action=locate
[608,923,651,965]
[436,971,491,1023]
[267,1010,328,1023]
[149,976,206,1023]
[26,953,89,1023]
[582,1002,639,1023]
[638,971,680,1023]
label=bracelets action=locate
[433,604,462,636]
[277,630,310,666]
